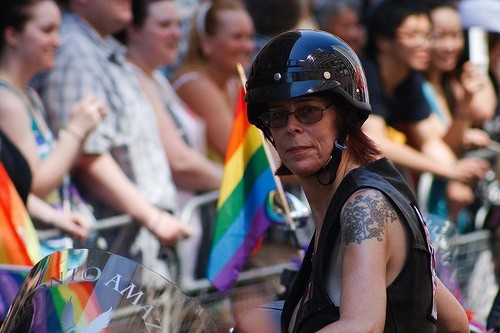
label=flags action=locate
[204,86,281,295]
[1,160,108,333]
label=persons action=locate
[1,1,500,333]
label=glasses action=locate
[435,31,462,39]
[402,31,432,41]
[256,102,336,129]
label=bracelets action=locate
[64,125,85,142]
[148,208,165,233]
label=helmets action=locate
[244,29,371,131]
